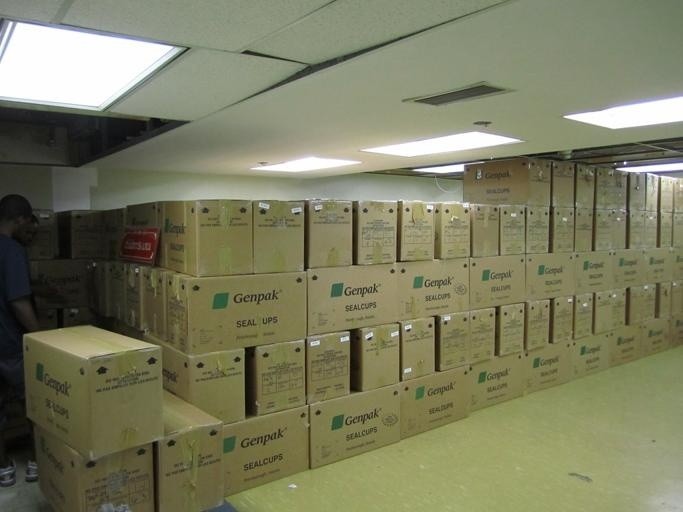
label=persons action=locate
[0,193,42,488]
[12,213,39,246]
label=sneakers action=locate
[24,456,40,483]
[0,455,17,488]
